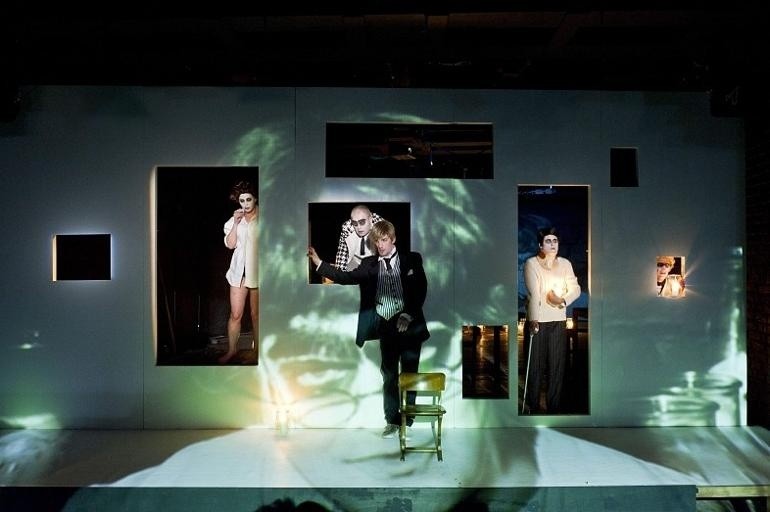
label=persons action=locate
[308,220,430,438]
[322,205,385,283]
[221,182,260,361]
[525,231,581,413]
[657,256,685,298]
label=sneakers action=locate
[399,426,413,442]
[382,423,399,439]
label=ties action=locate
[383,249,398,271]
[360,237,365,255]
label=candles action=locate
[672,282,679,296]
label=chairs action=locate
[397,371,448,461]
